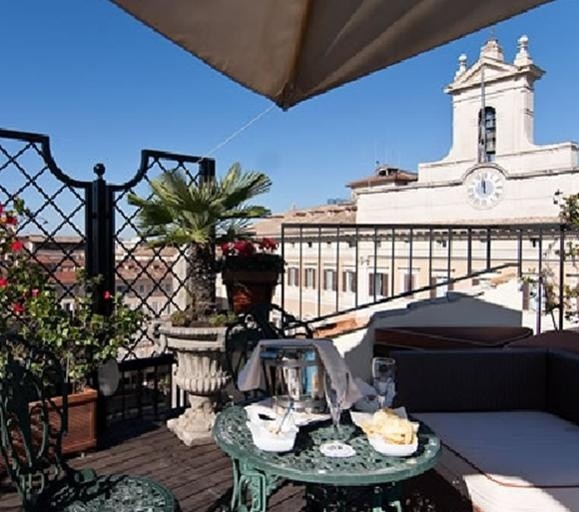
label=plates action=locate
[349,406,419,457]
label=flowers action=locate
[1,198,149,382]
[220,238,279,255]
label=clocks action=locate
[462,163,507,211]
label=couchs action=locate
[372,326,578,511]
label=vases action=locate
[219,254,284,319]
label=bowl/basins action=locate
[246,418,300,452]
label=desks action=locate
[212,395,442,510]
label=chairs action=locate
[2,334,179,511]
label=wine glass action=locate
[319,372,356,458]
[371,358,396,410]
